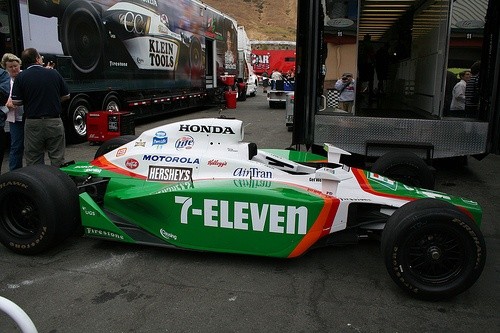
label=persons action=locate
[0,66,11,175]
[11,46,70,171]
[463,65,482,119]
[1,52,55,173]
[334,72,357,115]
[450,70,472,118]
[250,64,294,102]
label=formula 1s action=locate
[0,117,486,304]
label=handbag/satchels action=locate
[469,92,480,104]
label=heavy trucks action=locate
[1,0,250,144]
[249,40,298,84]
[292,0,497,179]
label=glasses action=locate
[41,56,44,61]
